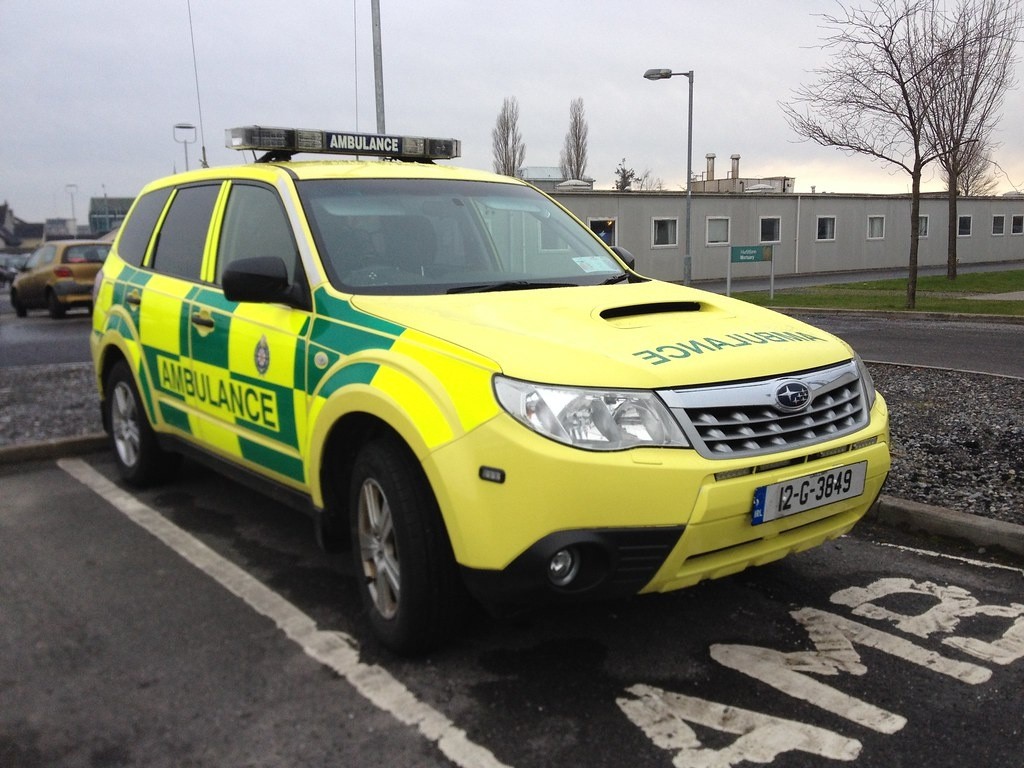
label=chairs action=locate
[378,215,449,286]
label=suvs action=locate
[90,125,890,635]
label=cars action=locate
[10,239,117,319]
[0,251,32,289]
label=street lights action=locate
[64,185,80,239]
[646,66,694,283]
[175,122,196,170]
